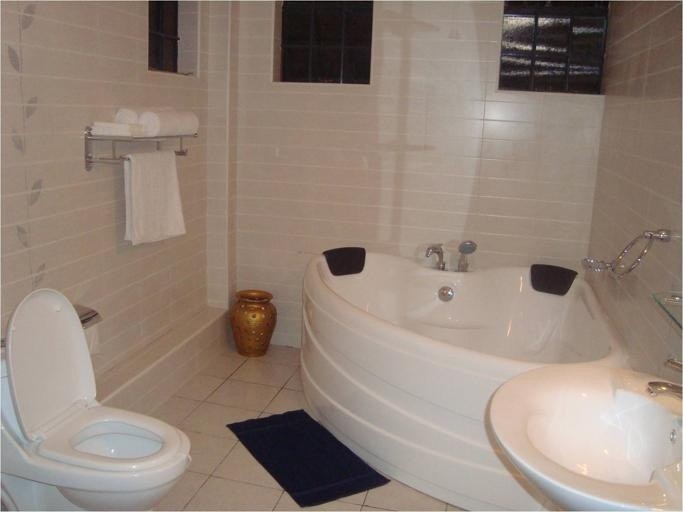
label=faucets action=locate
[426,244,445,269]
[646,381,683,399]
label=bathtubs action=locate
[301,251,626,512]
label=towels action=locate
[122,150,186,247]
[89,107,199,138]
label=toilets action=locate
[0,288,191,511]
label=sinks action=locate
[489,365,683,512]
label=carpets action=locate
[225,408,390,507]
